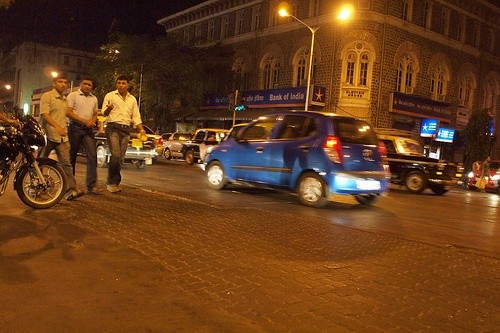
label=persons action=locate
[207,134,215,141]
[476,156,491,193]
[66,76,105,195]
[27,75,85,200]
[472,160,482,171]
[0,114,22,130]
[101,74,147,192]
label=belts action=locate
[107,123,129,129]
[71,121,87,128]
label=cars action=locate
[466,161,500,194]
[77,114,230,168]
[204,110,391,208]
[376,133,455,196]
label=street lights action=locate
[277,3,352,111]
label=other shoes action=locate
[88,187,104,194]
[481,190,485,192]
[107,184,122,193]
[66,188,85,200]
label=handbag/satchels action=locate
[475,177,486,190]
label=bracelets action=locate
[140,130,146,132]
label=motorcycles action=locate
[0,101,68,210]
